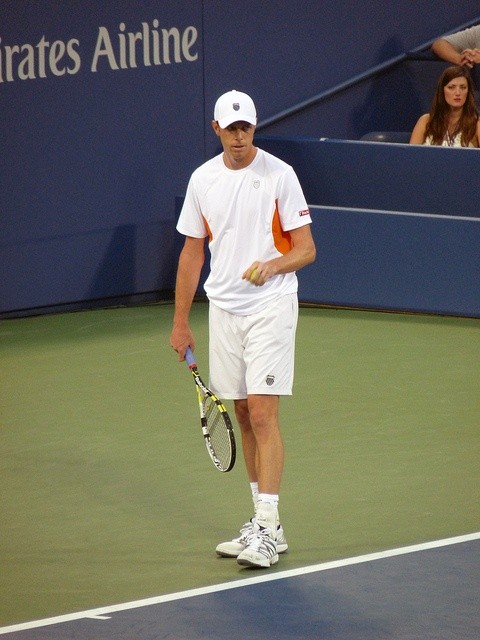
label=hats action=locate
[214,90,257,129]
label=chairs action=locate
[362,130,410,144]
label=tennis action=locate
[248,268,257,281]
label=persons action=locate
[170,89,317,568]
[434,22,480,69]
[409,64,479,148]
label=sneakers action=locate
[237,522,279,567]
[216,517,288,558]
